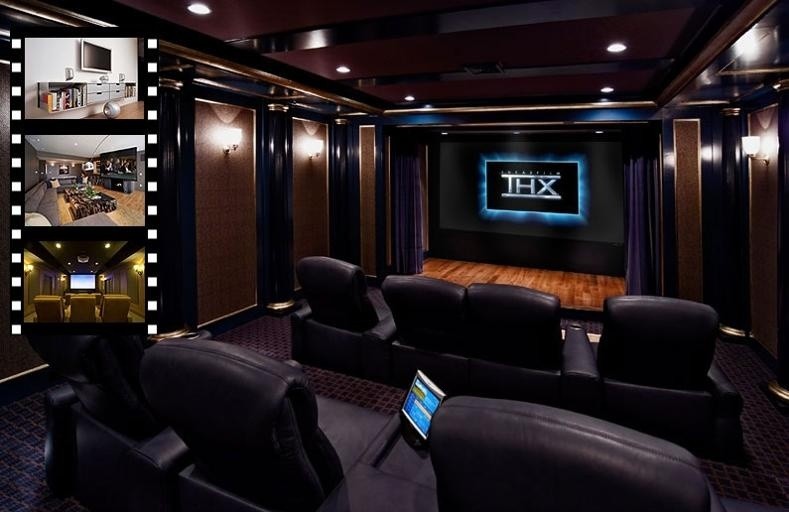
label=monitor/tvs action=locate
[69,274,98,293]
[399,370,446,442]
[100,147,138,181]
[80,40,112,74]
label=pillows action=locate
[45,178,61,189]
[76,175,83,184]
[82,176,88,184]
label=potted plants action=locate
[84,186,95,198]
[73,183,80,191]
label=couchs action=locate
[57,178,91,192]
[25,180,57,226]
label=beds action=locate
[61,212,118,226]
[69,192,117,221]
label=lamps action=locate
[222,128,243,155]
[307,140,324,160]
[742,135,774,167]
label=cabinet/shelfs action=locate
[35,81,137,114]
[104,178,135,194]
